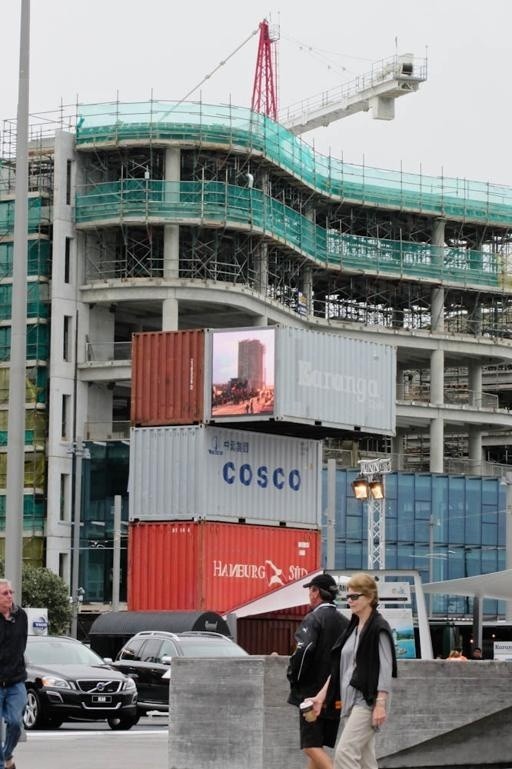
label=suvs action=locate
[114,630,249,717]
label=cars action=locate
[21,635,141,732]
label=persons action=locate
[470,647,487,660]
[286,572,351,768]
[392,628,398,652]
[447,647,468,660]
[0,578,28,768]
[301,571,399,769]
[214,384,275,415]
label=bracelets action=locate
[375,697,386,703]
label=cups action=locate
[299,701,316,723]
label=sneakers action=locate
[4,758,16,769]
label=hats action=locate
[302,575,338,594]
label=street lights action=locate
[349,457,392,581]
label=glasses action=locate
[346,593,365,600]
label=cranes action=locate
[158,10,429,142]
